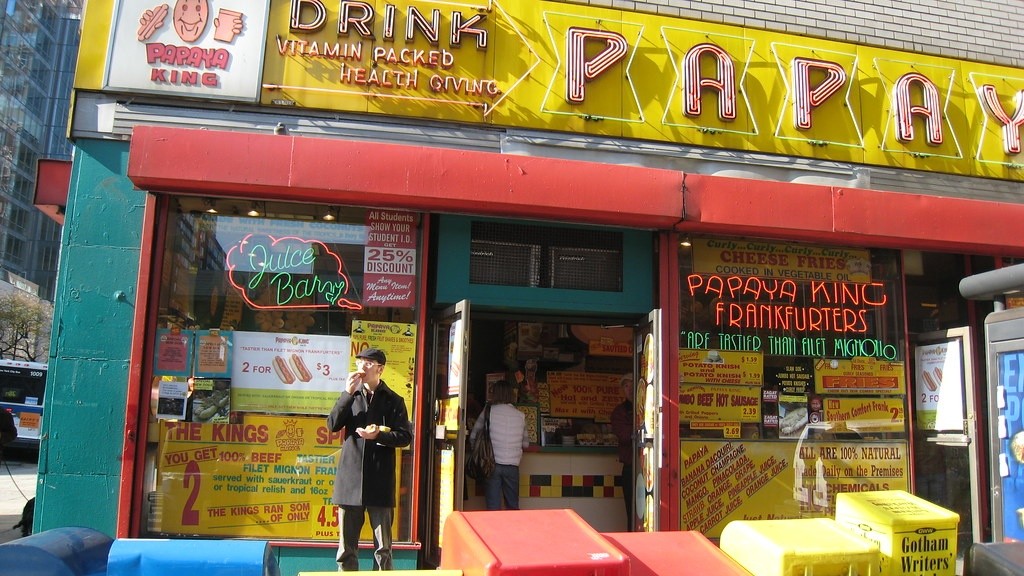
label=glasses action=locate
[356,359,381,369]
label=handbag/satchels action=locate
[464,401,495,478]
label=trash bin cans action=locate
[0,490,1024,576]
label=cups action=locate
[377,425,391,446]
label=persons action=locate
[611,372,635,533]
[469,379,529,512]
[327,348,413,571]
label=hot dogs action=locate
[922,367,944,391]
[779,407,808,435]
[194,390,230,419]
[273,354,313,384]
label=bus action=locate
[0,359,48,449]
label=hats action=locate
[619,373,634,384]
[354,348,387,364]
